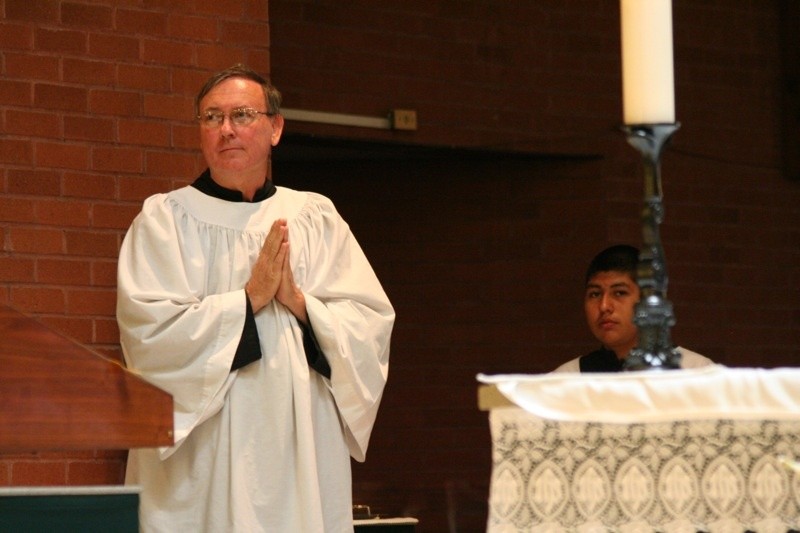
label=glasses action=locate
[197,108,274,128]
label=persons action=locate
[116,63,395,533]
[547,247,715,374]
